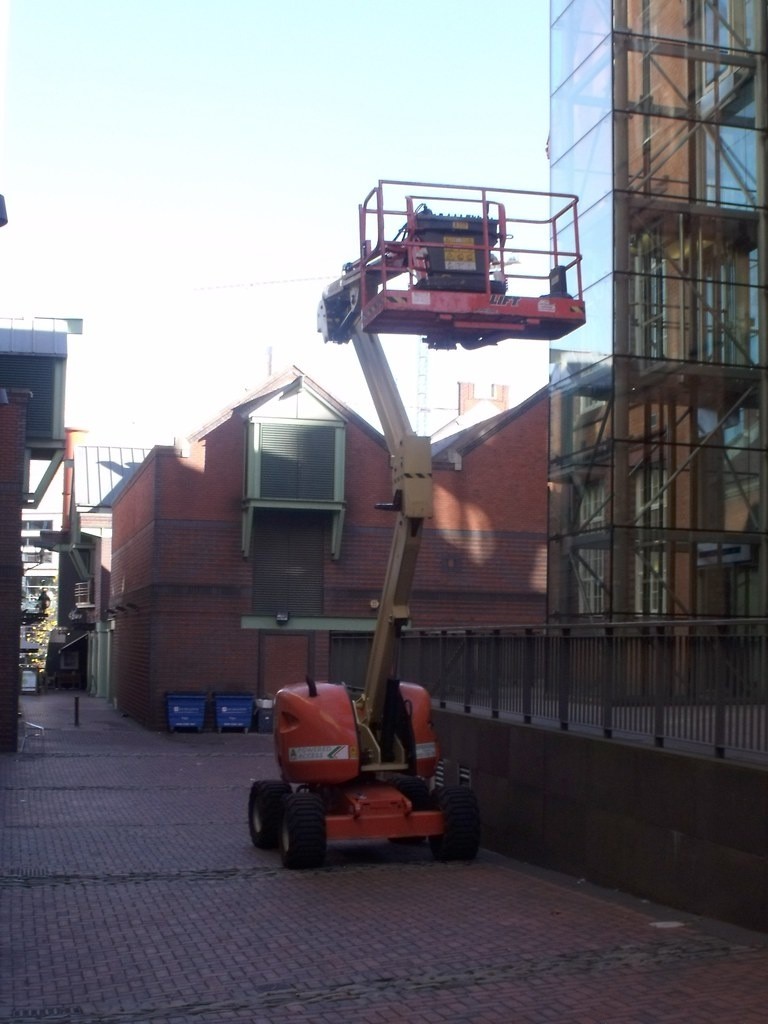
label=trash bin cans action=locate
[215,692,256,734]
[165,691,207,732]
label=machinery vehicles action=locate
[248,179,587,867]
